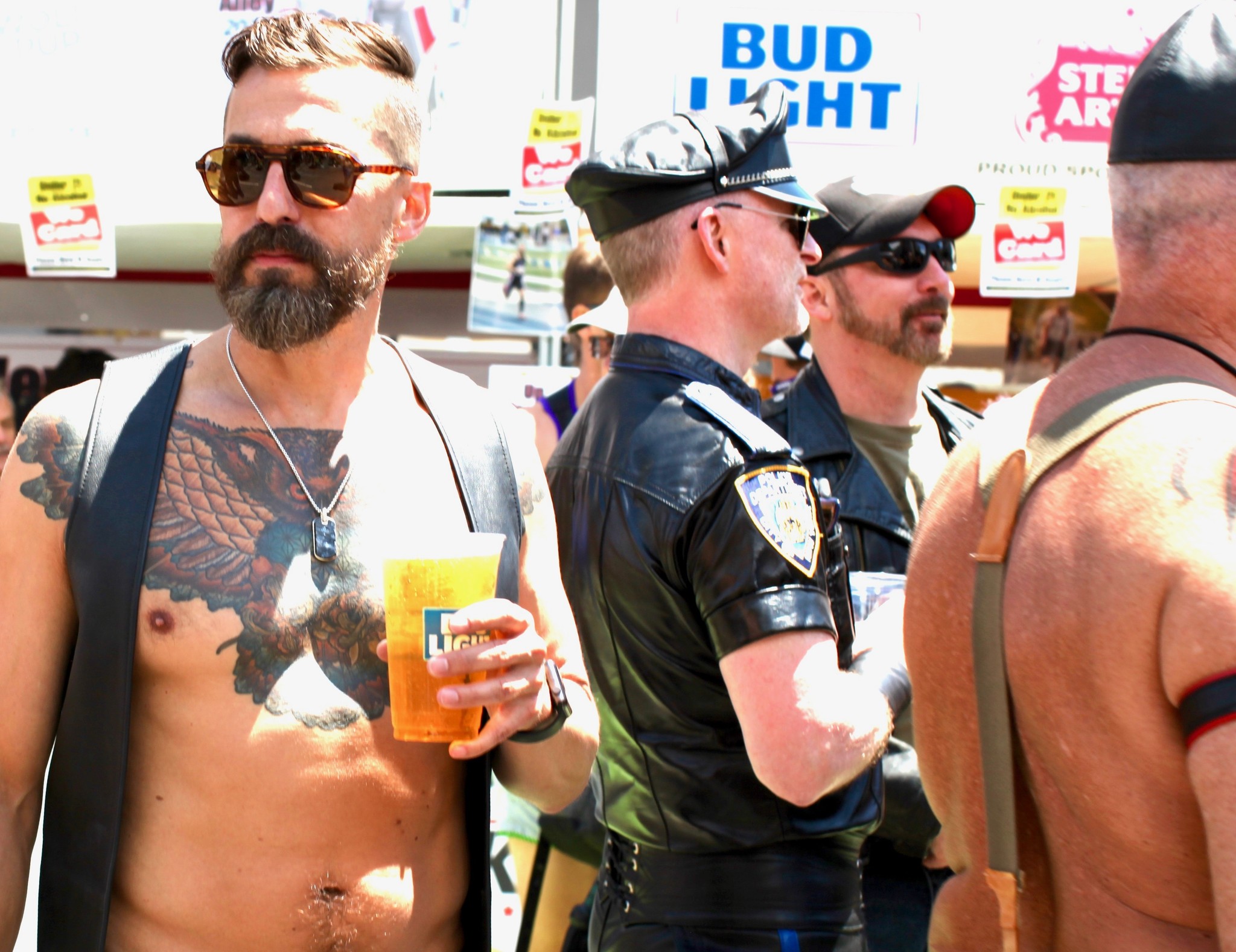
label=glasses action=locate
[812,236,956,274]
[195,144,416,210]
[691,201,812,252]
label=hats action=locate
[1106,0,1236,163]
[808,171,975,268]
[565,80,832,243]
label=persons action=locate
[501,242,528,322]
[0,13,603,952]
[897,0,1236,952]
[1032,304,1072,373]
[490,72,984,952]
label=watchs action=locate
[507,658,573,743]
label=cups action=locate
[847,569,907,628]
[377,530,507,743]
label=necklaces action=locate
[225,320,397,562]
